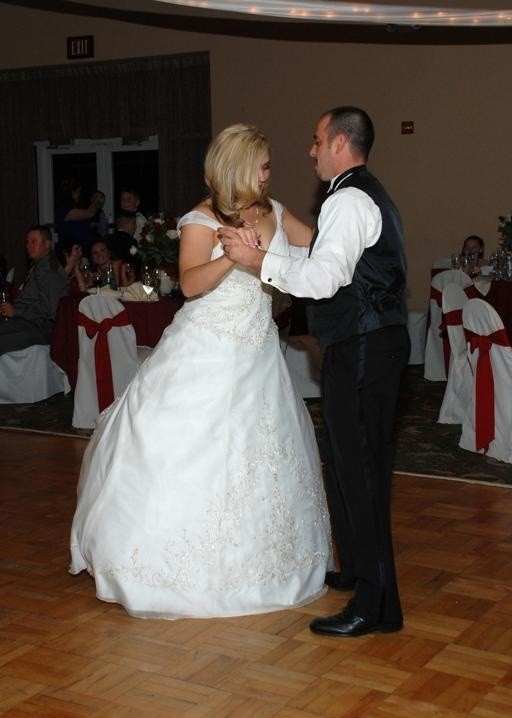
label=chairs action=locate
[279,295,321,399]
[0,268,154,430]
[424,257,512,465]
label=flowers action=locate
[133,217,178,281]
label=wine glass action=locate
[80,263,162,303]
[0,290,9,322]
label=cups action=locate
[450,253,475,269]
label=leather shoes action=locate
[325,571,355,590]
[310,609,402,638]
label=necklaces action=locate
[239,205,259,224]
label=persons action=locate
[464,235,490,266]
[0,182,154,357]
[221,107,413,638]
[68,121,336,621]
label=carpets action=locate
[0,367,512,488]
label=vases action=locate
[155,268,174,295]
